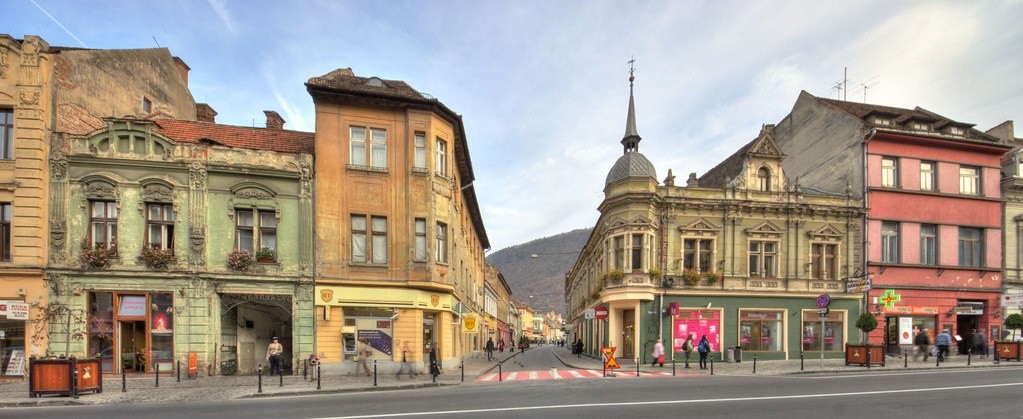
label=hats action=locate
[273,337,278,340]
[942,329,949,331]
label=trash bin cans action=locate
[571,344,578,354]
[728,346,742,363]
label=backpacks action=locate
[682,342,687,350]
[698,340,706,353]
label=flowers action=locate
[228,249,251,269]
[80,247,108,268]
[140,245,176,267]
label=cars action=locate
[93,346,113,359]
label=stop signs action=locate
[595,307,608,320]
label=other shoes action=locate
[704,368,708,370]
[685,366,691,368]
[269,372,272,376]
[368,372,372,376]
[701,367,703,369]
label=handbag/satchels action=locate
[657,355,664,364]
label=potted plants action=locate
[28,301,103,398]
[994,313,1023,361]
[256,247,274,263]
[844,311,886,368]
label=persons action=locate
[936,328,951,362]
[698,334,710,369]
[557,339,564,347]
[353,338,371,376]
[429,343,438,382]
[971,329,986,358]
[685,334,693,368]
[266,336,282,375]
[498,339,514,352]
[650,337,664,367]
[486,337,494,360]
[913,328,930,362]
[576,339,583,357]
